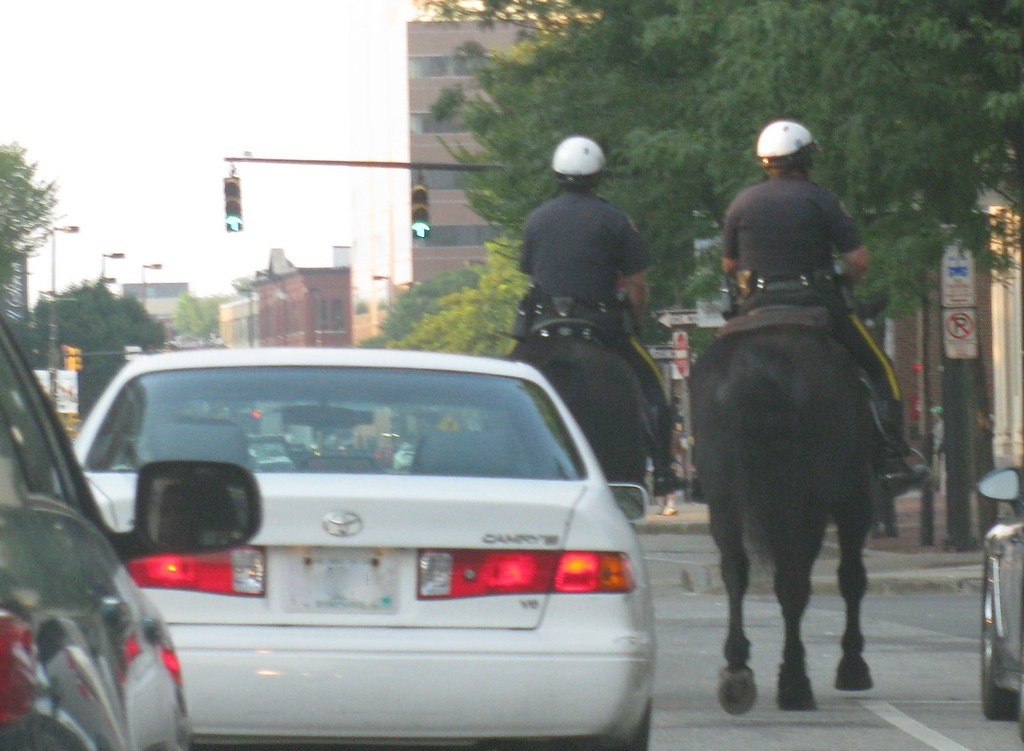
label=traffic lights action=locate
[410,181,431,241]
[223,174,243,234]
[65,344,84,371]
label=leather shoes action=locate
[652,476,689,497]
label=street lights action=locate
[49,226,80,293]
[140,263,165,309]
[372,273,394,309]
[99,252,125,284]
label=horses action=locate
[522,338,648,521]
[690,323,910,717]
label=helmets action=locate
[755,119,822,171]
[551,135,606,187]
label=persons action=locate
[684,120,932,496]
[511,136,691,496]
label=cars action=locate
[392,442,415,470]
[45,346,660,751]
[974,465,1024,737]
[0,312,265,751]
[371,447,394,471]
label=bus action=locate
[248,427,295,475]
